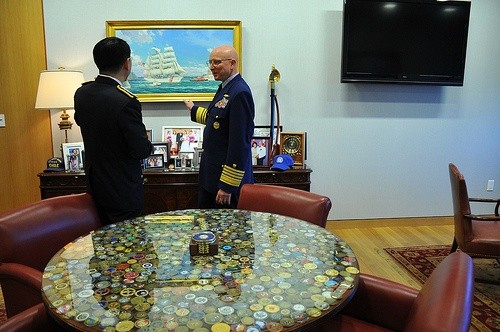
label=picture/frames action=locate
[62,141,84,173]
[253,125,283,152]
[105,20,243,104]
[251,136,271,169]
[279,132,305,166]
[140,125,204,170]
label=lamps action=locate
[35,67,85,157]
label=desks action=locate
[42,207,359,332]
[37,166,312,211]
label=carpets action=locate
[383,244,500,332]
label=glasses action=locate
[206,58,233,68]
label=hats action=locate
[271,154,294,170]
[43,157,65,171]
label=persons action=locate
[189,209,255,303]
[252,141,267,165]
[89,217,159,332]
[155,146,166,155]
[167,130,199,152]
[147,158,162,166]
[73,149,79,155]
[183,45,256,209]
[73,37,155,227]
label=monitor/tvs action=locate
[340,0,472,88]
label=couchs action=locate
[0,182,475,332]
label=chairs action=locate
[448,162,500,264]
